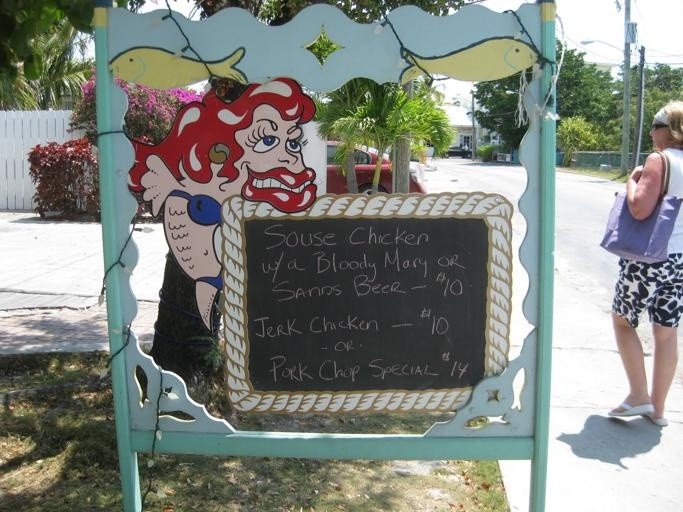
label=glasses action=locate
[651,124,668,131]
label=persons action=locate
[597,98,681,430]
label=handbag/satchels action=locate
[600,150,683,263]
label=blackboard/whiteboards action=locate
[221,191,513,413]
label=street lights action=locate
[504,89,524,100]
[579,38,648,180]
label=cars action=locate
[440,147,472,160]
[325,140,428,196]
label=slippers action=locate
[642,412,668,426]
[608,402,654,416]
